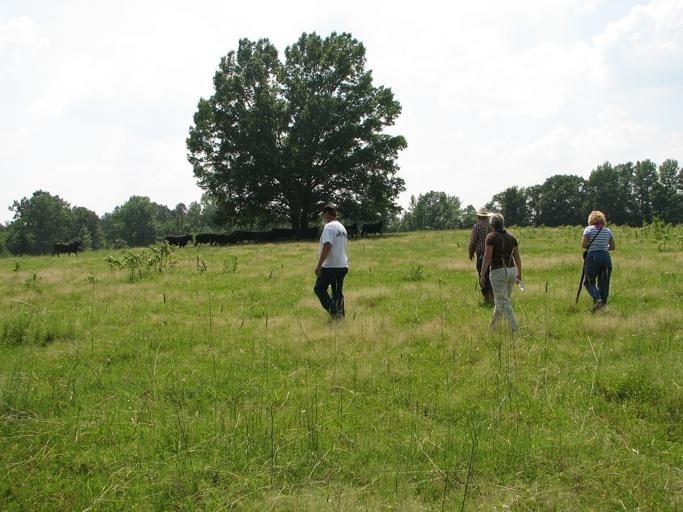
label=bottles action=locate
[515,279,527,292]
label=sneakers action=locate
[592,299,602,310]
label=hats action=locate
[318,205,337,216]
[476,207,492,217]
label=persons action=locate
[479,213,526,337]
[313,204,348,323]
[467,206,493,307]
[580,210,615,313]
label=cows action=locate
[164,234,192,248]
[51,240,81,258]
[360,220,385,237]
[192,223,361,248]
